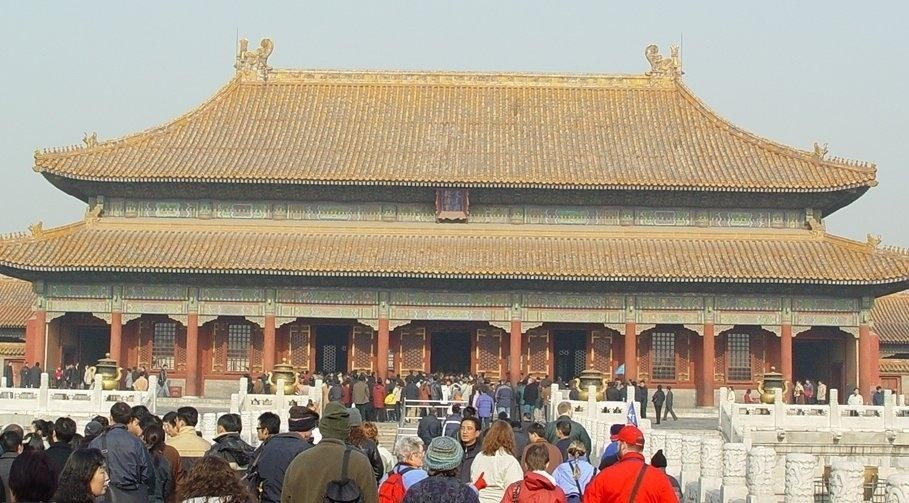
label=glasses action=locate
[256,427,265,430]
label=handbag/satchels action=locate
[321,450,364,503]
[99,431,149,503]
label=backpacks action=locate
[377,466,413,502]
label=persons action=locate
[66,432,86,447]
[583,425,677,502]
[500,444,565,503]
[481,411,521,437]
[163,412,202,439]
[744,389,753,414]
[30,362,43,387]
[652,384,665,425]
[51,448,109,503]
[597,423,625,471]
[520,412,534,430]
[377,436,487,503]
[554,421,577,463]
[0,431,23,502]
[347,407,384,486]
[816,381,826,413]
[321,369,378,421]
[176,453,254,503]
[88,402,154,503]
[650,449,683,501]
[464,369,564,418]
[237,369,315,397]
[521,423,563,473]
[281,402,378,503]
[794,379,803,405]
[8,451,57,503]
[165,406,211,457]
[378,372,416,420]
[128,405,151,436]
[402,436,480,503]
[442,405,464,437]
[55,363,172,400]
[417,406,442,450]
[550,441,600,502]
[4,361,15,398]
[92,416,108,428]
[30,420,57,450]
[662,386,678,421]
[603,378,649,418]
[873,386,884,415]
[83,421,103,445]
[847,387,863,416]
[19,362,32,387]
[364,420,394,473]
[544,402,591,456]
[803,379,814,405]
[45,417,77,488]
[244,413,280,494]
[256,406,319,503]
[140,425,173,503]
[727,386,735,402]
[416,371,470,412]
[135,416,182,477]
[452,417,482,482]
[470,420,524,503]
[22,432,45,451]
[0,424,23,455]
[203,414,256,487]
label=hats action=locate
[347,407,362,426]
[288,406,318,432]
[610,425,644,449]
[650,449,668,468]
[84,421,103,436]
[319,402,351,441]
[424,435,464,471]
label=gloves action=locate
[474,472,487,491]
